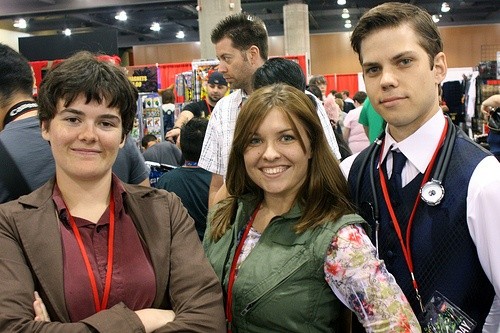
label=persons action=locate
[481,94,500,162]
[204,83,421,333]
[0,51,226,333]
[307,76,386,161]
[338,4,500,333]
[0,44,229,244]
[198,15,342,246]
[213,58,319,205]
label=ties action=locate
[388,151,406,214]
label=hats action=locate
[207,72,227,86]
